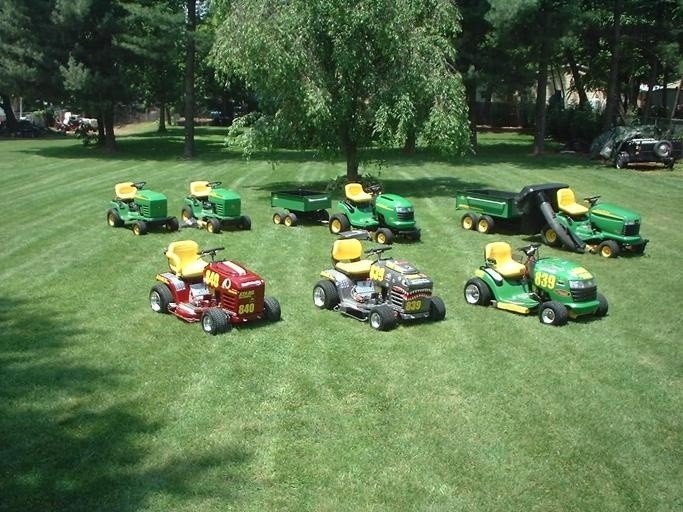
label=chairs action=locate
[555,186,590,218]
[112,182,139,201]
[344,181,371,206]
[483,240,528,279]
[328,237,375,278]
[188,178,215,200]
[166,240,208,279]
